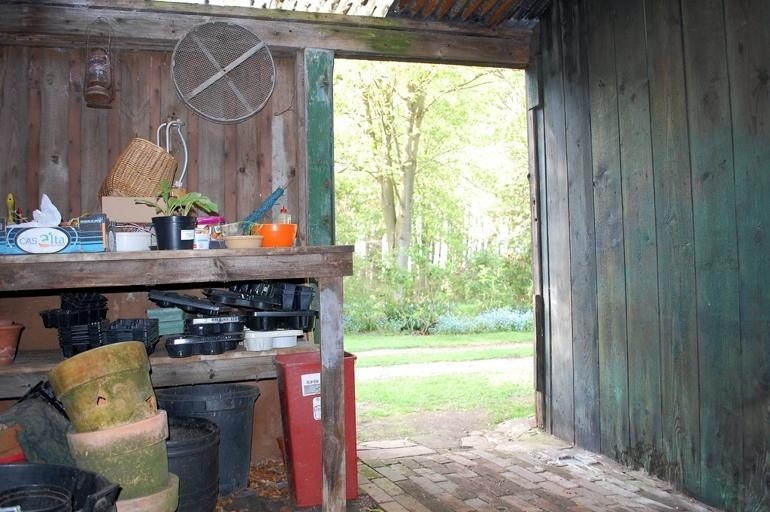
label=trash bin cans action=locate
[271,350,360,510]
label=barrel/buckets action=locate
[153,383,260,497]
[166,414,219,512]
[0,463,122,512]
[1,485,71,512]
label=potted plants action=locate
[133,179,219,251]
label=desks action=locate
[2,244,356,512]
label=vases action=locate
[1,318,261,512]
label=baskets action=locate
[98,138,178,197]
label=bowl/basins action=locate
[223,236,263,248]
[251,223,298,248]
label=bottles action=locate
[278,208,291,224]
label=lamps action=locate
[84,17,114,105]
